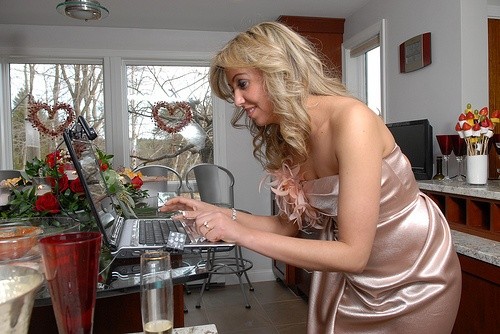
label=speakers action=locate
[385,119,433,180]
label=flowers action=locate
[4,149,143,224]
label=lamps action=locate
[55,0,110,23]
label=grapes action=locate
[467,104,485,120]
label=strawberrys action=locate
[455,107,500,131]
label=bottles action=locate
[433,156,445,180]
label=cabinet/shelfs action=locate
[268,15,345,288]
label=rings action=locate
[204,221,209,228]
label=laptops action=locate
[62,129,237,259]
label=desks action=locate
[0,195,211,334]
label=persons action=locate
[161,22,462,334]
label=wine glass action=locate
[435,135,459,183]
[451,137,467,182]
[492,134,500,180]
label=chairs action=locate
[133,165,255,313]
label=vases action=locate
[466,155,488,184]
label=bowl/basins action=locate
[0,265,45,334]
[0,226,41,261]
[0,217,81,264]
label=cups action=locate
[140,252,174,334]
[39,232,103,334]
[466,155,488,184]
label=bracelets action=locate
[232,208,237,220]
